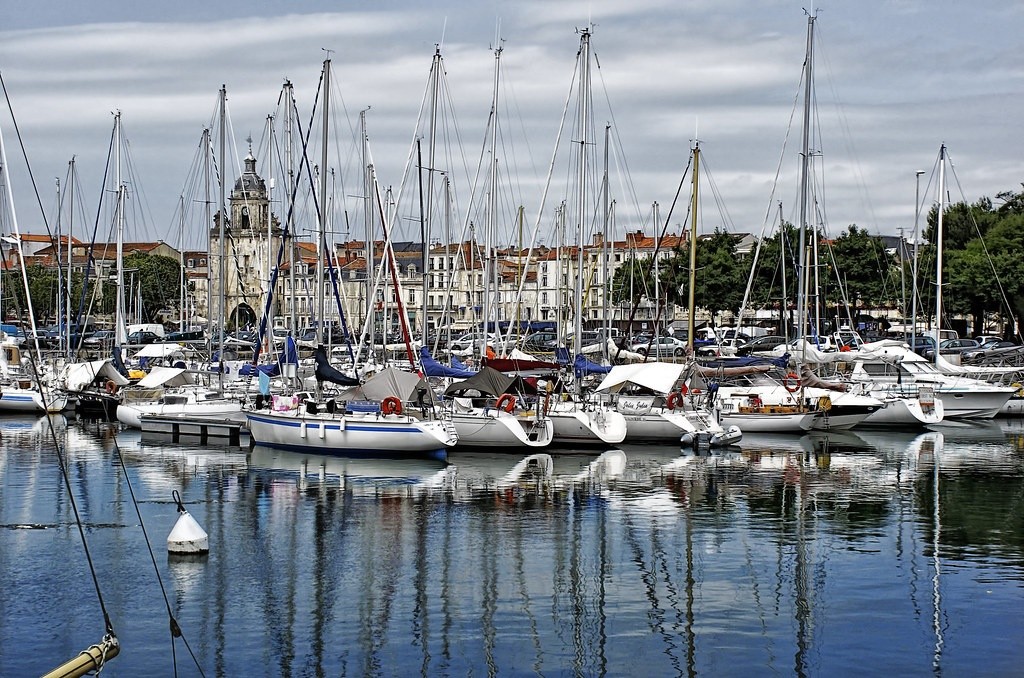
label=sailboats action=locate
[0,0,1024,454]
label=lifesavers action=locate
[496,393,515,413]
[784,374,800,392]
[106,380,116,394]
[841,346,850,352]
[382,396,401,415]
[667,393,685,409]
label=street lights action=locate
[911,168,926,353]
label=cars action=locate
[82,331,116,349]
[959,340,1024,364]
[899,336,936,357]
[14,330,48,349]
[515,332,558,351]
[698,337,747,357]
[271,329,291,350]
[922,338,982,362]
[628,336,688,358]
[734,336,790,357]
[481,334,526,354]
[446,331,497,351]
[544,331,602,349]
[127,330,162,350]
[427,334,463,352]
[975,335,1005,346]
[681,338,715,352]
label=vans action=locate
[922,329,959,344]
[44,323,93,350]
